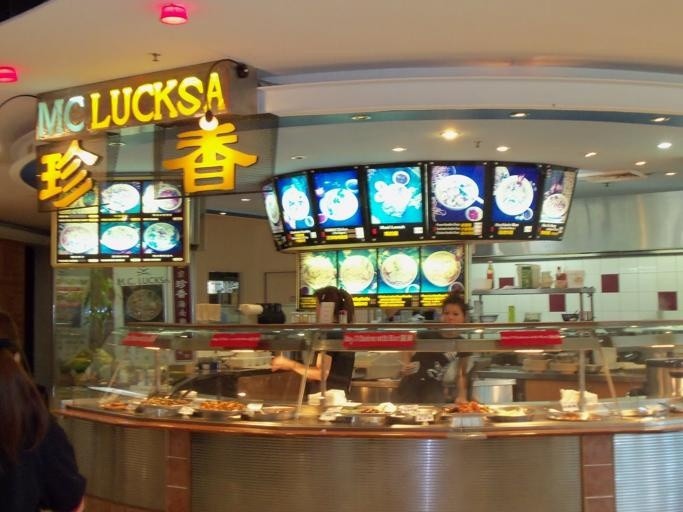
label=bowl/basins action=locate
[582,362,602,373]
[522,358,552,371]
[478,314,498,322]
[554,362,579,372]
[561,313,580,321]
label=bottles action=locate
[337,310,347,324]
[539,271,553,288]
[520,266,533,288]
[507,304,515,322]
[554,265,568,288]
[486,260,496,289]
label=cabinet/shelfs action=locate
[50,318,683,512]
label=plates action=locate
[494,173,533,220]
[319,178,358,221]
[301,251,460,293]
[57,181,182,254]
[434,174,484,222]
[263,190,280,225]
[541,192,568,219]
[393,171,410,186]
[125,288,162,321]
[282,185,309,220]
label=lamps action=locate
[199,58,248,131]
[0,66,18,83]
[161,4,188,25]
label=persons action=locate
[271,286,356,401]
[0,309,87,512]
[389,291,472,404]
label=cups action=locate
[399,309,414,323]
